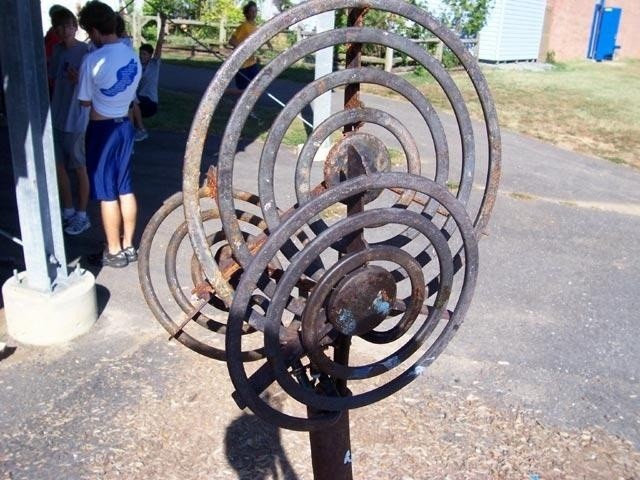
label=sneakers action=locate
[133,128,148,142]
[87,241,138,268]
[62,207,91,235]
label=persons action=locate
[218,0,260,120]
[40,11,93,237]
[86,12,134,54]
[125,10,169,143]
[74,0,144,270]
[43,3,77,100]
